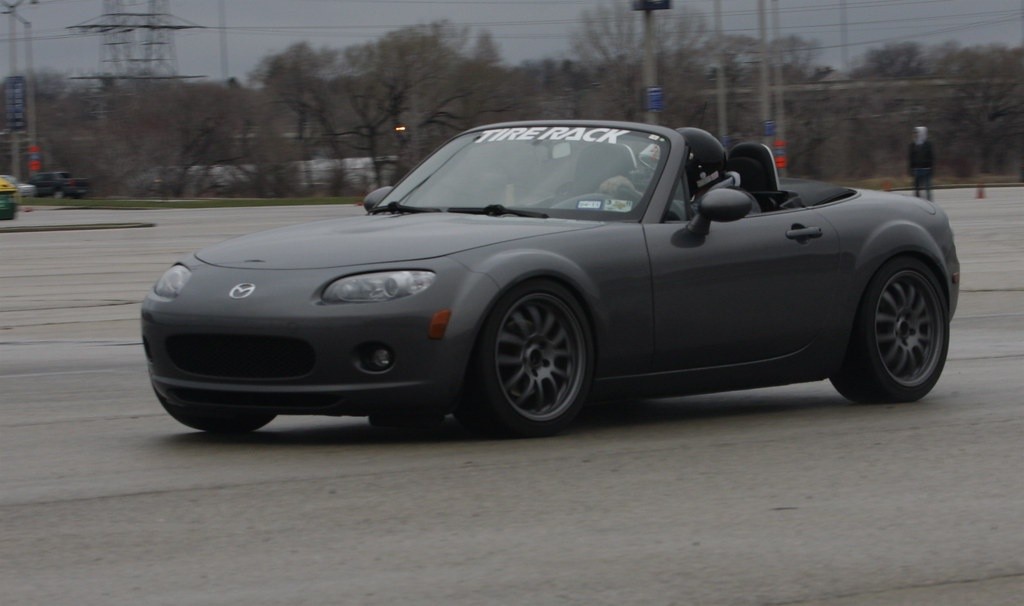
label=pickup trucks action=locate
[28,172,90,199]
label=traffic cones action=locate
[975,187,985,197]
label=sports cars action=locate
[138,120,960,438]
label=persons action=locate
[600,128,761,220]
[908,126,936,201]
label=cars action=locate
[0,177,19,203]
[0,174,37,199]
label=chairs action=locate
[573,142,636,193]
[727,142,782,196]
